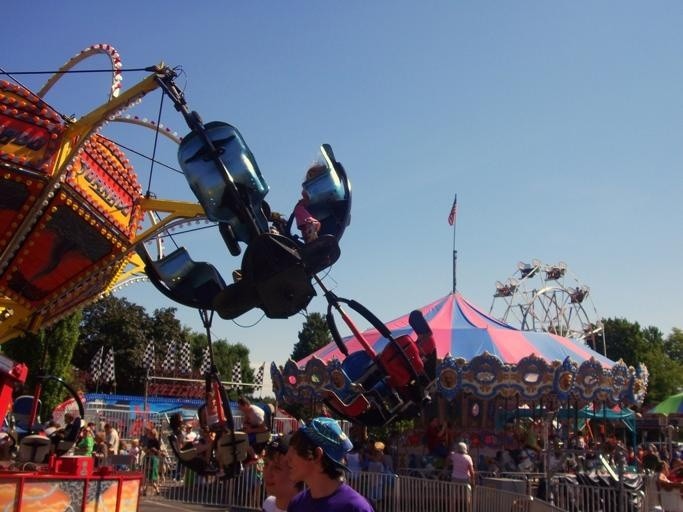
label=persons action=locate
[170,398,269,468]
[349,417,474,484]
[231,225,279,283]
[262,418,374,511]
[596,420,606,442]
[44,414,163,496]
[663,420,681,441]
[528,416,541,429]
[477,431,683,511]
[505,417,514,444]
[558,418,569,440]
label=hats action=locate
[302,417,353,472]
[457,442,468,454]
[374,442,384,451]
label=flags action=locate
[231,358,242,390]
[178,338,190,375]
[89,346,102,385]
[161,336,175,374]
[142,338,155,370]
[198,344,211,376]
[254,363,263,391]
[447,197,456,225]
[102,345,115,384]
